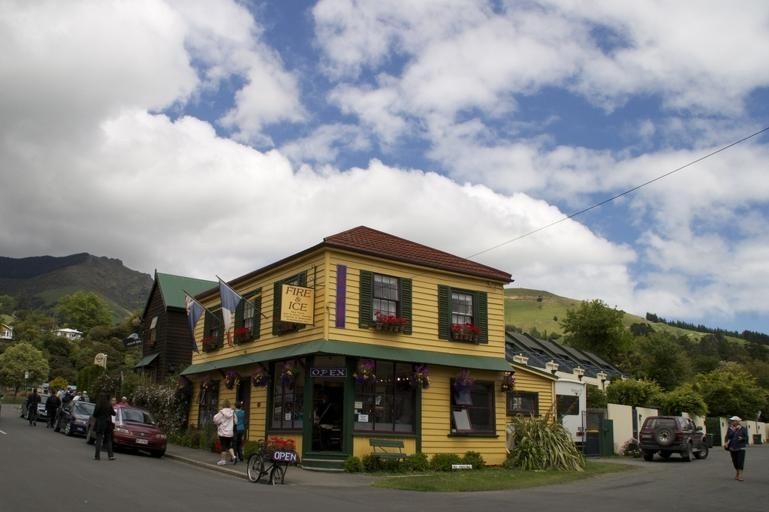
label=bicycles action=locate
[247,439,289,488]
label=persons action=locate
[230,401,246,462]
[18,384,131,460]
[212,397,238,465]
[723,415,748,481]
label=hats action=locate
[729,415,741,421]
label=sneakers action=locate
[231,456,237,465]
[216,460,226,465]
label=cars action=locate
[85,404,167,458]
[18,394,50,421]
[53,400,98,436]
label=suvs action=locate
[639,416,710,462]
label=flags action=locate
[217,278,241,346]
[183,292,204,354]
[137,271,166,338]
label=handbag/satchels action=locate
[233,412,238,441]
